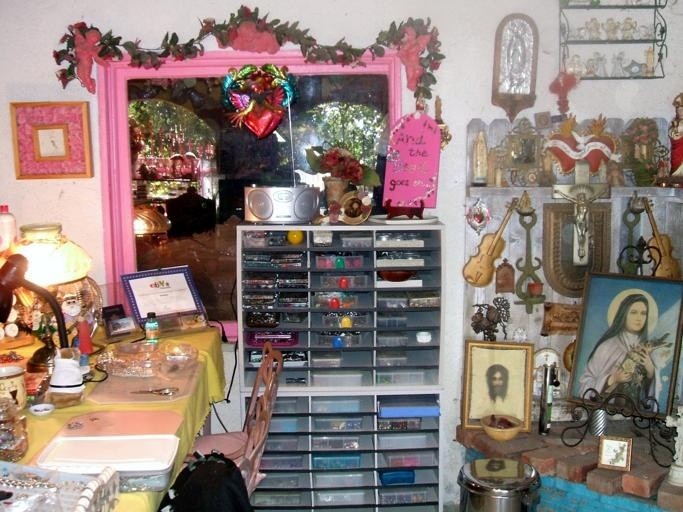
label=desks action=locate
[0,318,226,512]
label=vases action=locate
[321,176,349,211]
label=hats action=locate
[607,288,659,336]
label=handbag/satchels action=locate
[156,452,255,512]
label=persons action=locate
[555,189,606,257]
[471,363,517,419]
[577,288,675,413]
[668,93,683,188]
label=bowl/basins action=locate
[480,415,524,440]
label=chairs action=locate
[184,342,283,501]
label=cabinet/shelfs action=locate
[558,0,668,80]
[236,220,446,512]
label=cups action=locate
[416,332,431,343]
[0,366,28,410]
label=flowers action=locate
[304,145,381,188]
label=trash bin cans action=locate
[455,458,543,512]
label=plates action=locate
[30,404,54,415]
[96,341,197,378]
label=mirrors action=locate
[96,48,402,312]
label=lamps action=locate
[0,222,92,287]
[133,204,173,235]
[0,253,86,393]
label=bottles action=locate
[589,410,607,439]
[145,311,159,344]
[0,204,15,254]
[645,45,656,77]
[132,182,169,270]
[0,398,29,462]
[70,319,92,365]
[538,363,555,437]
[472,130,504,187]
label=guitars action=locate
[463,198,518,287]
[641,197,680,279]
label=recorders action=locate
[244,184,320,224]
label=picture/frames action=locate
[10,101,93,180]
[565,272,683,421]
[597,434,632,472]
[461,340,534,434]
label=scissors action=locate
[130,387,179,397]
